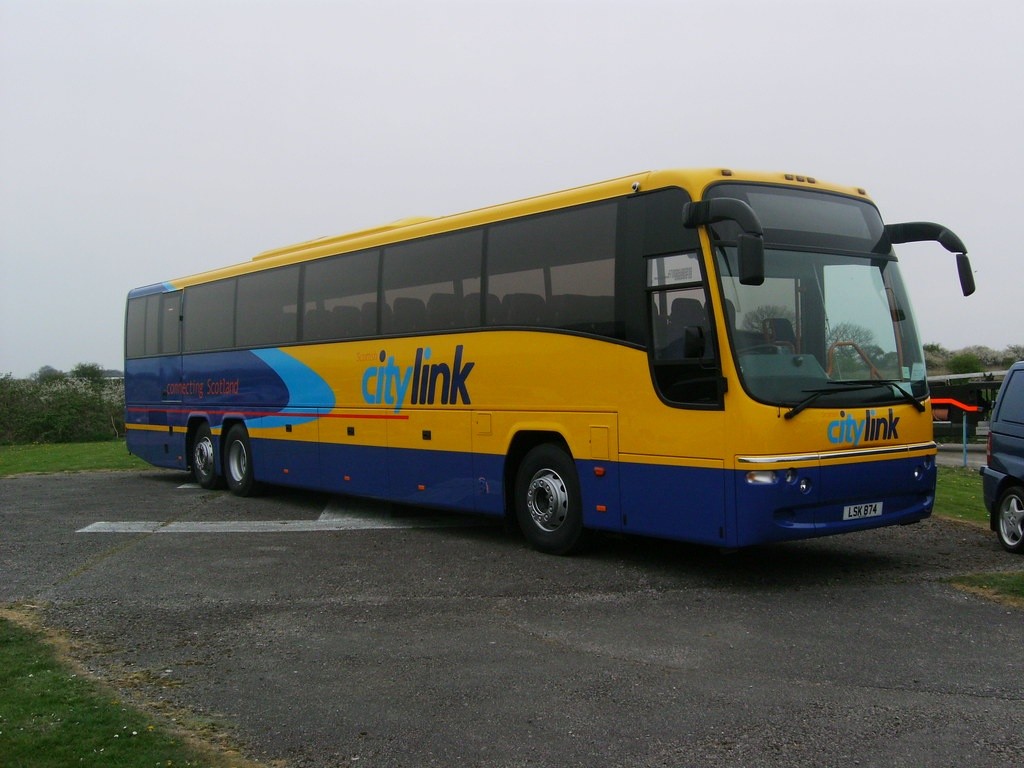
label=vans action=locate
[979,360,1024,554]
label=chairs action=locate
[282,294,735,345]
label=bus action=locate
[122,166,976,556]
